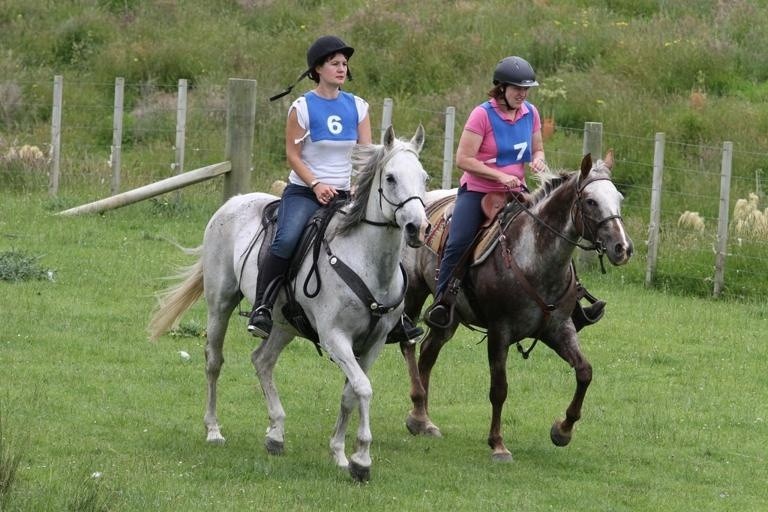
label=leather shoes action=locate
[572,301,606,332]
[424,306,448,327]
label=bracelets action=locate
[309,179,321,190]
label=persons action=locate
[419,56,605,334]
[247,35,423,343]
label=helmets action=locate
[494,55,539,87]
[307,36,354,80]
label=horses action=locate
[397,149,634,461]
[145,123,432,484]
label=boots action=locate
[386,319,424,344]
[249,248,291,332]
